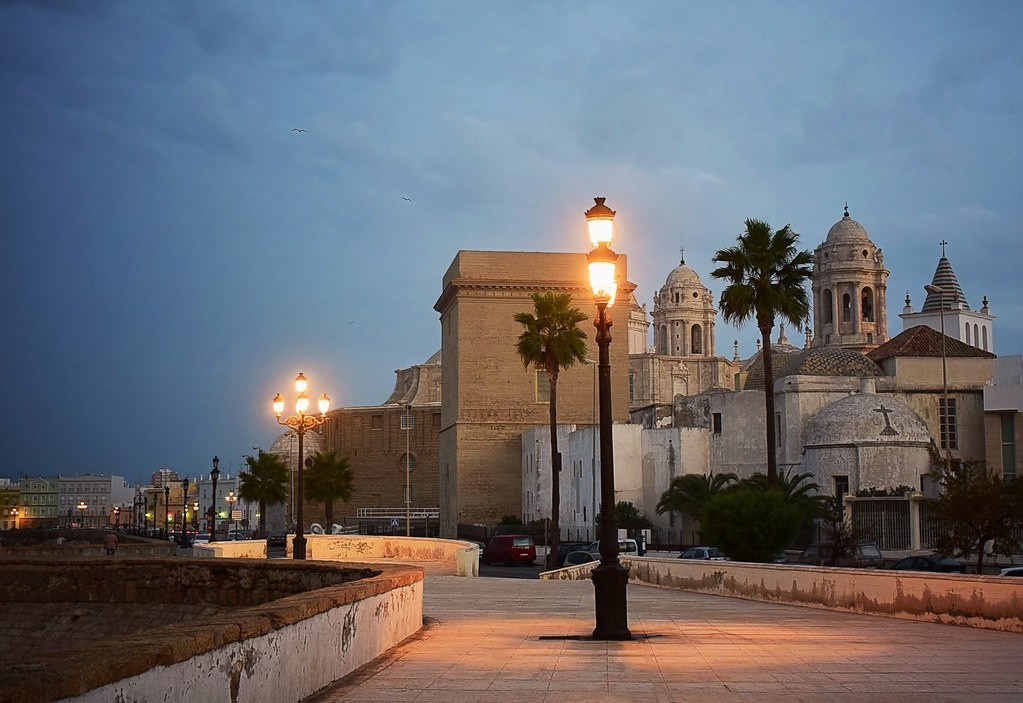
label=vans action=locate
[483,534,537,566]
[545,537,638,570]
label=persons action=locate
[104,531,118,556]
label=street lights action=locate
[128,491,158,538]
[583,196,635,639]
[113,506,119,528]
[77,501,87,528]
[10,508,19,529]
[182,476,189,533]
[164,486,170,541]
[209,456,220,543]
[225,491,237,523]
[271,373,331,559]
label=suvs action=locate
[796,538,886,569]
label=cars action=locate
[998,565,1023,578]
[888,555,966,573]
[475,542,486,560]
[675,546,730,560]
[168,529,198,541]
[267,531,287,546]
[194,533,208,545]
[226,531,254,540]
